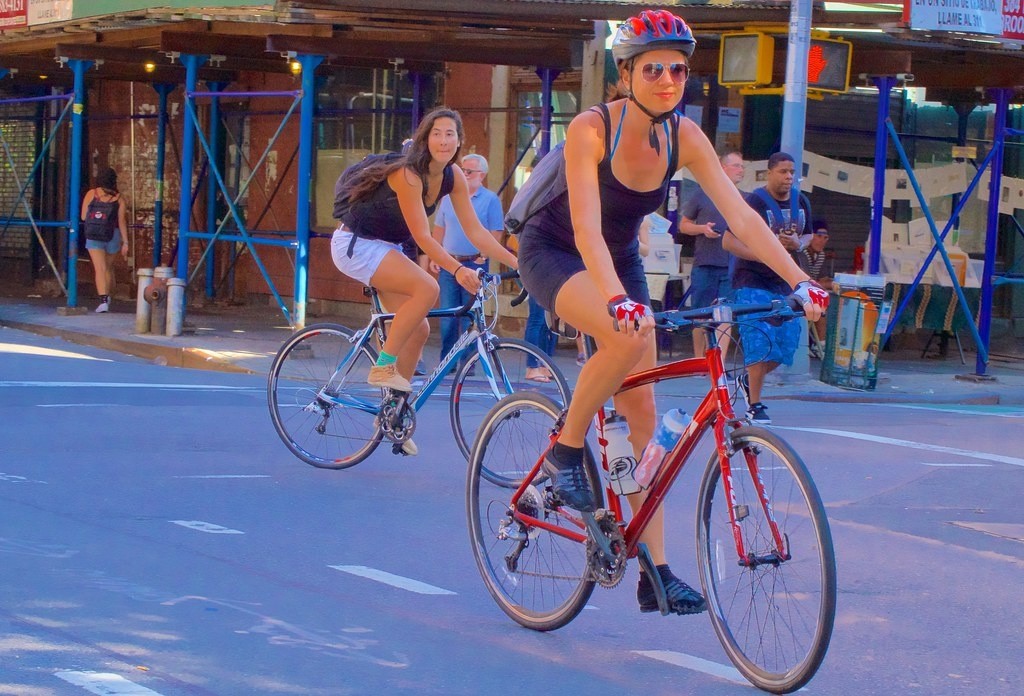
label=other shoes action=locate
[809,338,825,357]
[726,372,735,384]
[415,360,426,374]
[95,294,111,312]
[576,353,586,367]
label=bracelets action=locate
[453,264,466,277]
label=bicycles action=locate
[267,268,572,490]
[468,299,838,695]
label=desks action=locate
[867,247,980,364]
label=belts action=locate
[451,253,481,262]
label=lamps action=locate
[290,61,302,76]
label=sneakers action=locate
[637,574,707,611]
[373,415,418,456]
[745,405,772,424]
[540,445,597,512]
[367,364,413,392]
[736,373,750,407]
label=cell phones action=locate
[713,229,721,234]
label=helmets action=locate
[612,9,697,70]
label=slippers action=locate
[525,375,552,383]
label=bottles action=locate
[603,410,641,496]
[635,407,691,490]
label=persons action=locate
[678,151,751,385]
[517,6,832,616]
[720,152,815,424]
[429,152,505,375]
[796,220,831,357]
[330,107,519,456]
[80,167,129,311]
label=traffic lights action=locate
[718,32,774,88]
[807,37,853,94]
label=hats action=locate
[815,218,832,234]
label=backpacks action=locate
[332,153,408,259]
[503,107,680,242]
[83,193,121,242]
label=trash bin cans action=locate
[820,273,888,391]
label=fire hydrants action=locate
[144,266,174,334]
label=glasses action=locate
[461,167,482,174]
[633,63,691,84]
